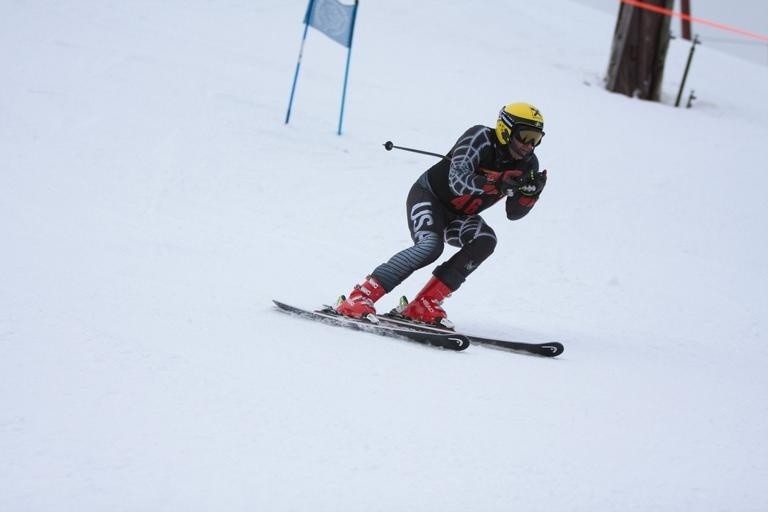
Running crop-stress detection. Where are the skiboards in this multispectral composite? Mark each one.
[272,299,564,357]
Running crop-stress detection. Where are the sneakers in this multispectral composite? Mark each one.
[335,294,379,325]
[401,299,455,330]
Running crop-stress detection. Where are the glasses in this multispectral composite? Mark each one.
[512,123,544,147]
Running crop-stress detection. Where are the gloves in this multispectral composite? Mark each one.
[521,171,548,196]
[494,170,522,198]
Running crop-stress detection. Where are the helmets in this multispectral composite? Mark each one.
[495,102,544,155]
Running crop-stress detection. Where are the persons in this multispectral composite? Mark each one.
[337,101,547,325]
